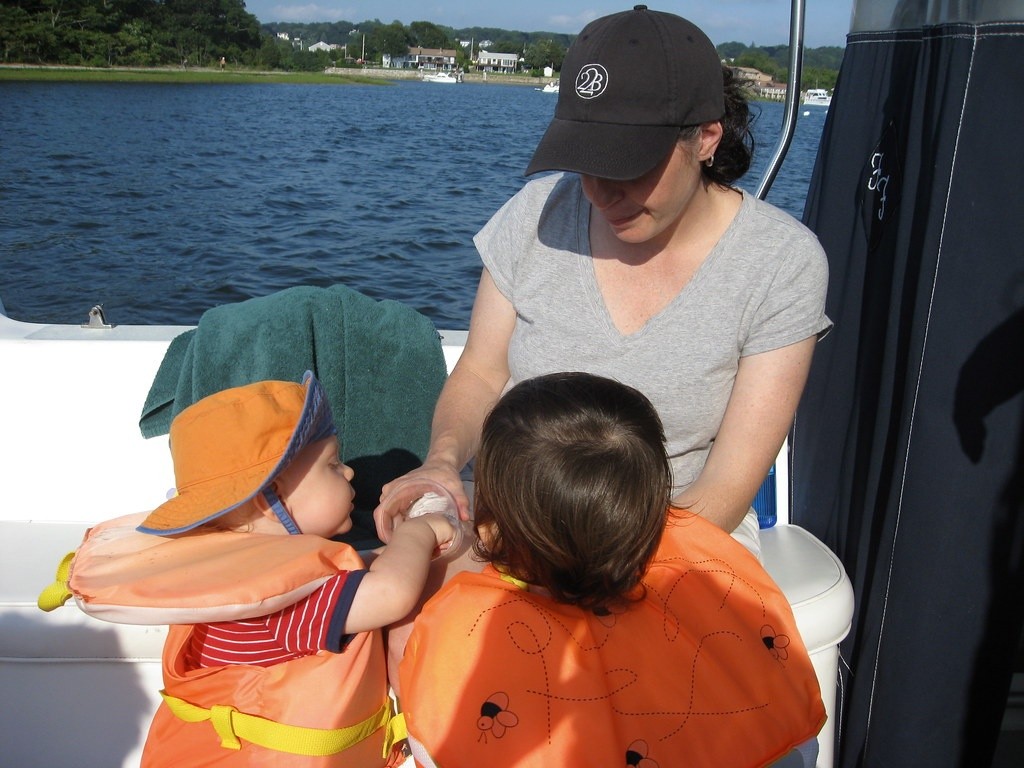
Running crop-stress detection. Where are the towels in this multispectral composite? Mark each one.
[139,285,449,552]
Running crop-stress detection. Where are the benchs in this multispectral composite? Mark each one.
[0,519,855,768]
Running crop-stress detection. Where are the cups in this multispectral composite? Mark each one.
[381,479,463,562]
[750,461,777,529]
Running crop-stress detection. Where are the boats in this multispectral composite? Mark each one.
[804,88,831,106]
[541,81,561,95]
[423,70,458,83]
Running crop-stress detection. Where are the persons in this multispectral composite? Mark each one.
[388,368,828,768]
[36,369,456,768]
[372,4,835,561]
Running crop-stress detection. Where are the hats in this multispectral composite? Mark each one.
[135,370,338,537]
[525,4,726,180]
